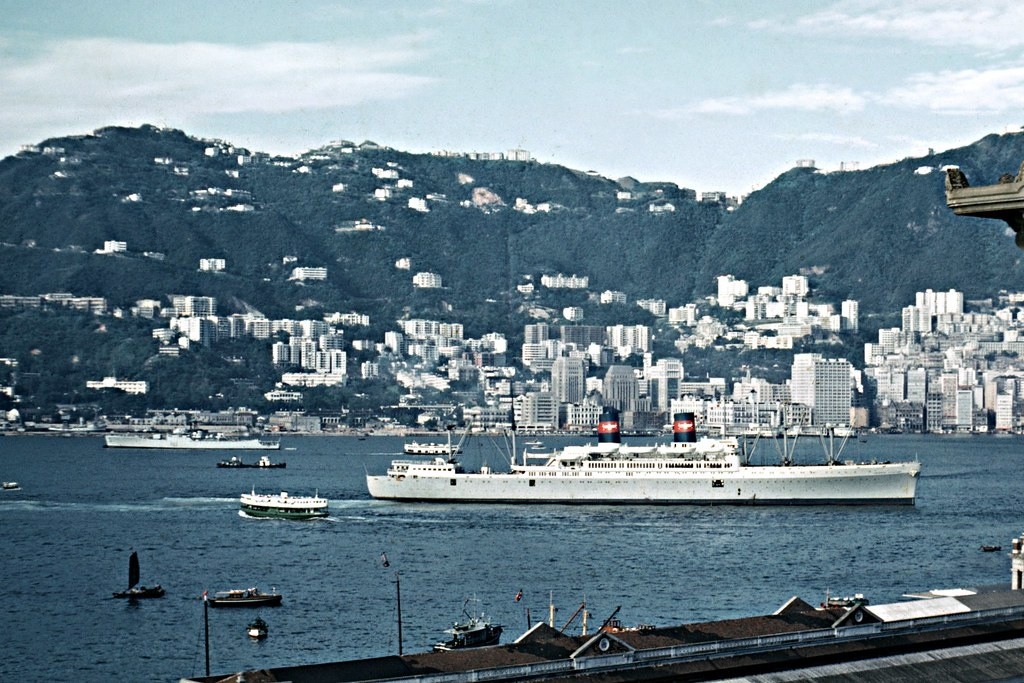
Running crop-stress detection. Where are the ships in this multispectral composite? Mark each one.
[363,406,921,505]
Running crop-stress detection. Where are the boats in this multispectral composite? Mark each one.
[901,588,977,599]
[3,482,19,489]
[696,424,1024,438]
[599,618,655,633]
[404,440,464,455]
[530,445,547,450]
[432,592,506,651]
[240,483,330,520]
[982,544,1003,551]
[247,619,268,637]
[828,593,869,606]
[521,441,544,446]
[208,588,283,606]
[105,418,282,450]
[217,459,286,467]
[589,428,660,437]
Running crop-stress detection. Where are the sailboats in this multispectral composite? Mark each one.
[113,551,165,598]
